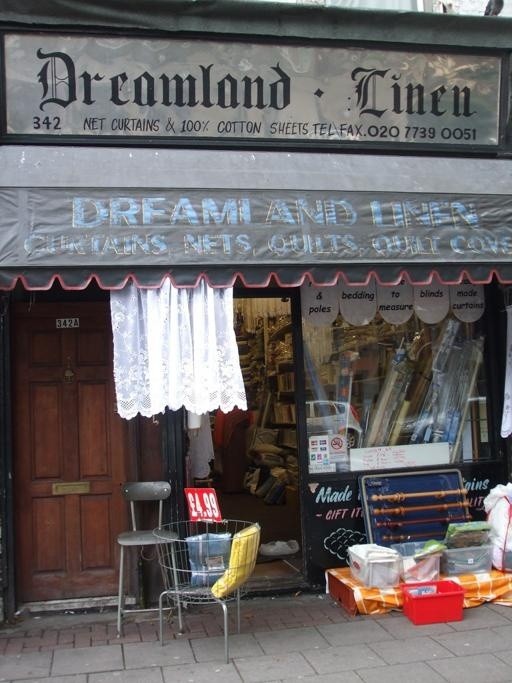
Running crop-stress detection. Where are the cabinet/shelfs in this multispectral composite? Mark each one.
[235,305,494,464]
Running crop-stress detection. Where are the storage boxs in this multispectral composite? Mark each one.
[440,536,493,577]
[347,543,400,589]
[390,541,439,583]
[400,580,466,625]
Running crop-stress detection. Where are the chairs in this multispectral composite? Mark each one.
[116,480,185,637]
